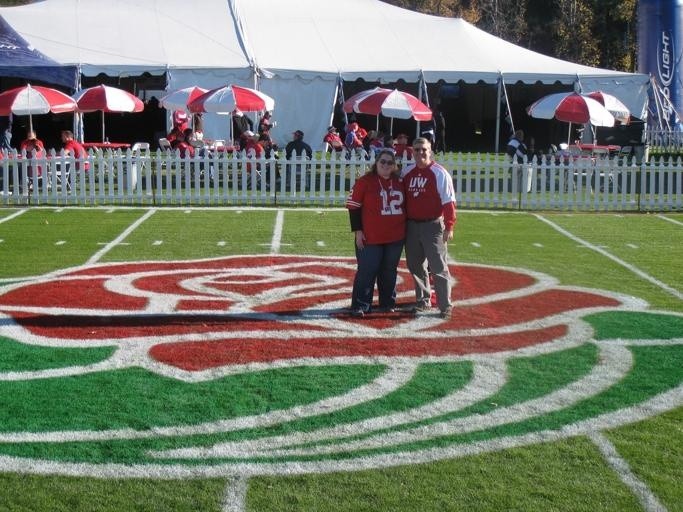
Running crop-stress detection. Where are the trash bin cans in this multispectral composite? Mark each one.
[117,156,142,191]
[510,161,533,193]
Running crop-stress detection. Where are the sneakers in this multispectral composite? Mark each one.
[440,310,451,319]
[382,306,394,313]
[351,307,364,317]
[412,305,431,313]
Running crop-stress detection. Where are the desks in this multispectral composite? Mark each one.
[538,143,620,192]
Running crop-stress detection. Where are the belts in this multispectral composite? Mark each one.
[406,218,436,223]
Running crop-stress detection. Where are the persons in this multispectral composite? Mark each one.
[24,139,42,179]
[402,137,458,320]
[0,120,15,154]
[581,124,597,147]
[230,107,280,189]
[321,121,412,160]
[60,130,90,191]
[20,129,44,150]
[506,129,528,159]
[285,130,313,167]
[347,149,407,320]
[165,111,213,180]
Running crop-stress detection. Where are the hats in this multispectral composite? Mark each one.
[291,130,304,136]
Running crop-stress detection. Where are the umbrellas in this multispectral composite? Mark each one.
[186,83,275,147]
[581,91,631,145]
[524,90,616,150]
[342,86,392,131]
[341,88,433,136]
[157,85,209,135]
[0,84,77,138]
[71,82,145,146]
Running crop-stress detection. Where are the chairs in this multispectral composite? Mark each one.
[613,146,632,166]
[47,137,310,192]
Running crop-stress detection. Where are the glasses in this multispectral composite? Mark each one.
[379,159,393,165]
